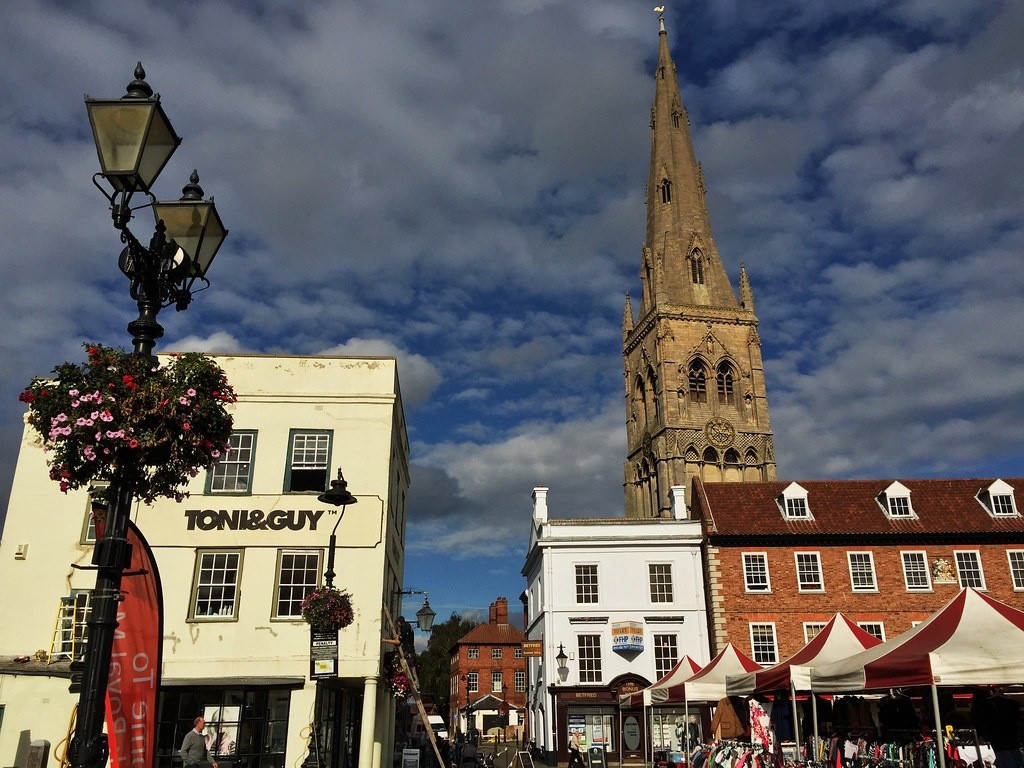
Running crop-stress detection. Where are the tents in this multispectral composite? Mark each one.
[619,586,1024,768]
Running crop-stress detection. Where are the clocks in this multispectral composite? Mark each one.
[704,416,736,449]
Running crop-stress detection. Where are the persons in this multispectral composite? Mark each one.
[180,717,219,768]
[675,714,699,753]
[398,616,414,657]
[745,398,752,424]
[568,731,587,768]
[707,338,714,355]
[411,726,480,768]
[678,393,685,419]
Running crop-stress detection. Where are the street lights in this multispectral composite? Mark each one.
[460,673,470,742]
[302,468,358,768]
[502,683,509,743]
[58,56,232,768]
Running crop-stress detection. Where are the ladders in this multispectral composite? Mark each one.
[47,597,77,665]
[384,603,446,768]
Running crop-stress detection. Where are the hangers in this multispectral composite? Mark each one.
[717,738,773,758]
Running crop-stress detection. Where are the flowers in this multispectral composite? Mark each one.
[377,672,412,702]
[16,339,238,505]
[299,585,356,633]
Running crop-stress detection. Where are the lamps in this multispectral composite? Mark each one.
[556,640,569,668]
[393,585,437,632]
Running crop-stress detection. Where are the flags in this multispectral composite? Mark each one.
[92,502,164,768]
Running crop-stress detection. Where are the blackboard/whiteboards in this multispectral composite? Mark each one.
[515,751,534,768]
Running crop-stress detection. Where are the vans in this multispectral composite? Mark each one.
[411,714,449,742]
